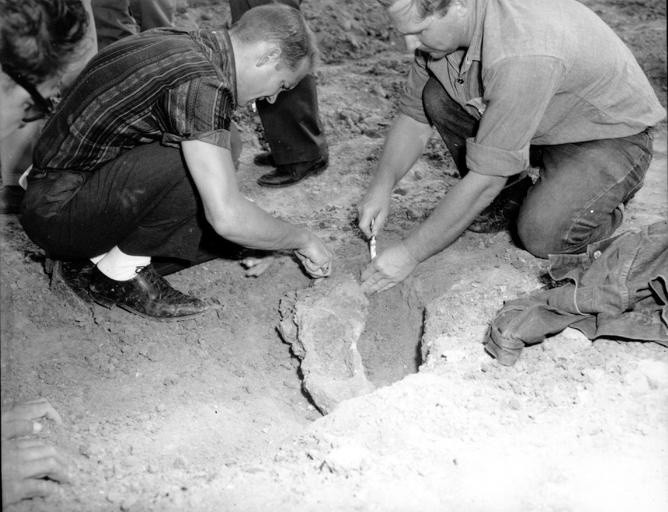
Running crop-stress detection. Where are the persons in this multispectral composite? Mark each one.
[230,0,331,188]
[0,0,177,212]
[353,0,668,299]
[16,2,337,321]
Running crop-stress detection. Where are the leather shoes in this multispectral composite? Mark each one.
[254,149,329,188]
[86,262,210,322]
